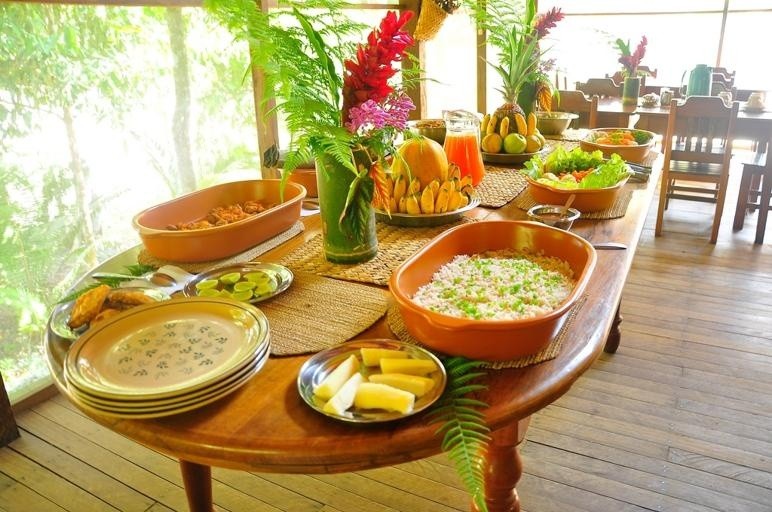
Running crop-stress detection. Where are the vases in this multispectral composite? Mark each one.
[516,82,538,124]
[313,146,379,266]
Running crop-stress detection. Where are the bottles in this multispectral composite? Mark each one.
[660,90,671,106]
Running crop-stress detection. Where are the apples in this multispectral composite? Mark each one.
[482,129,546,154]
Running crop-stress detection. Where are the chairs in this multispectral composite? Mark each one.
[550,66,772,245]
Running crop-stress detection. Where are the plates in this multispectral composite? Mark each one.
[64,300,271,422]
[49,287,175,343]
[367,189,483,226]
[480,143,549,165]
[294,337,452,424]
[180,263,302,303]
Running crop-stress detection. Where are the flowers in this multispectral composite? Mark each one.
[612,34,657,80]
[461,0,566,118]
[201,0,451,250]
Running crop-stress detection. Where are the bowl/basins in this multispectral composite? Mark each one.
[534,110,580,136]
[407,119,456,143]
[526,203,582,233]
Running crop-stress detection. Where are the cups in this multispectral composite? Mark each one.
[716,90,767,110]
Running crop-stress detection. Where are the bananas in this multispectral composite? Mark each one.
[377,162,474,215]
[481,113,537,138]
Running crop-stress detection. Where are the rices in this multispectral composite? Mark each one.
[407,247,577,320]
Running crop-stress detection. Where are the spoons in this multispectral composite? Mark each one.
[90,270,178,286]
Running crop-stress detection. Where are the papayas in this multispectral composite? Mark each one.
[391,136,449,192]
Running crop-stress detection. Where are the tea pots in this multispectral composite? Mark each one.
[680,64,713,99]
[441,109,486,188]
